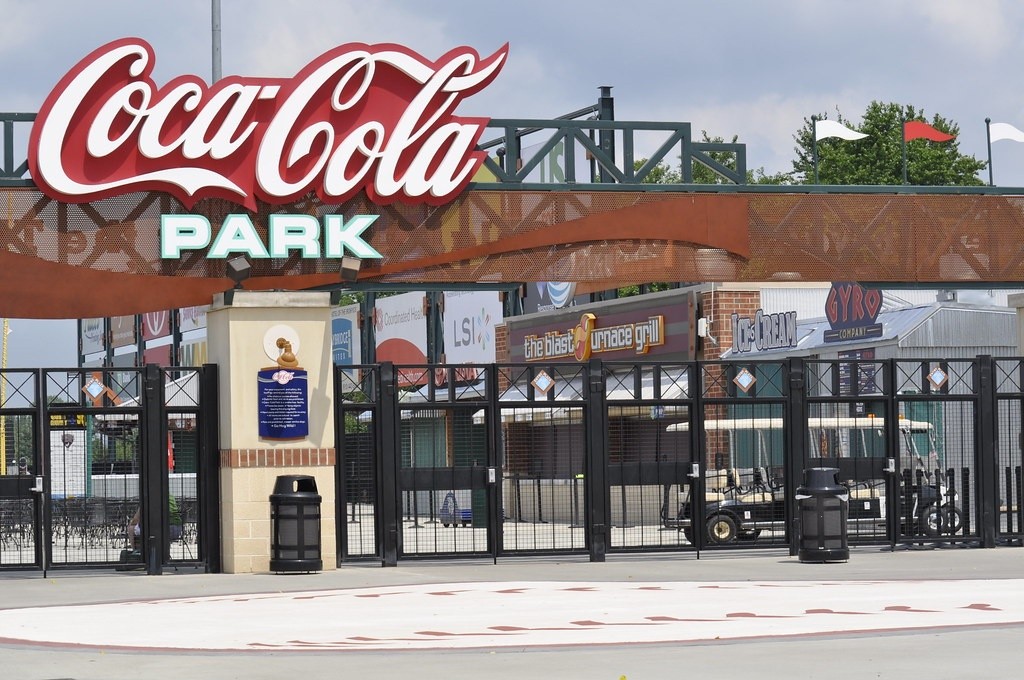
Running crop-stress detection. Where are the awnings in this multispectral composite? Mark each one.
[355,381,485,422]
[472,365,705,425]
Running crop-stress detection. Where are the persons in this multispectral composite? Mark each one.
[127,496,182,551]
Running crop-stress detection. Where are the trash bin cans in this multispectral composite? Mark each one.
[795,467,850,563]
[269,474,323,575]
